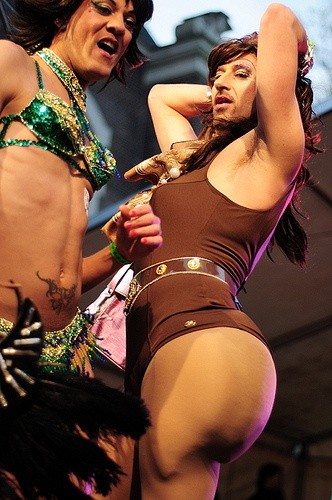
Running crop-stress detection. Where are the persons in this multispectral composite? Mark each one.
[0,0,163,500]
[123,3,314,500]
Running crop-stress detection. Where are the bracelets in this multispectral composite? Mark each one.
[301,40,315,63]
[109,241,134,266]
